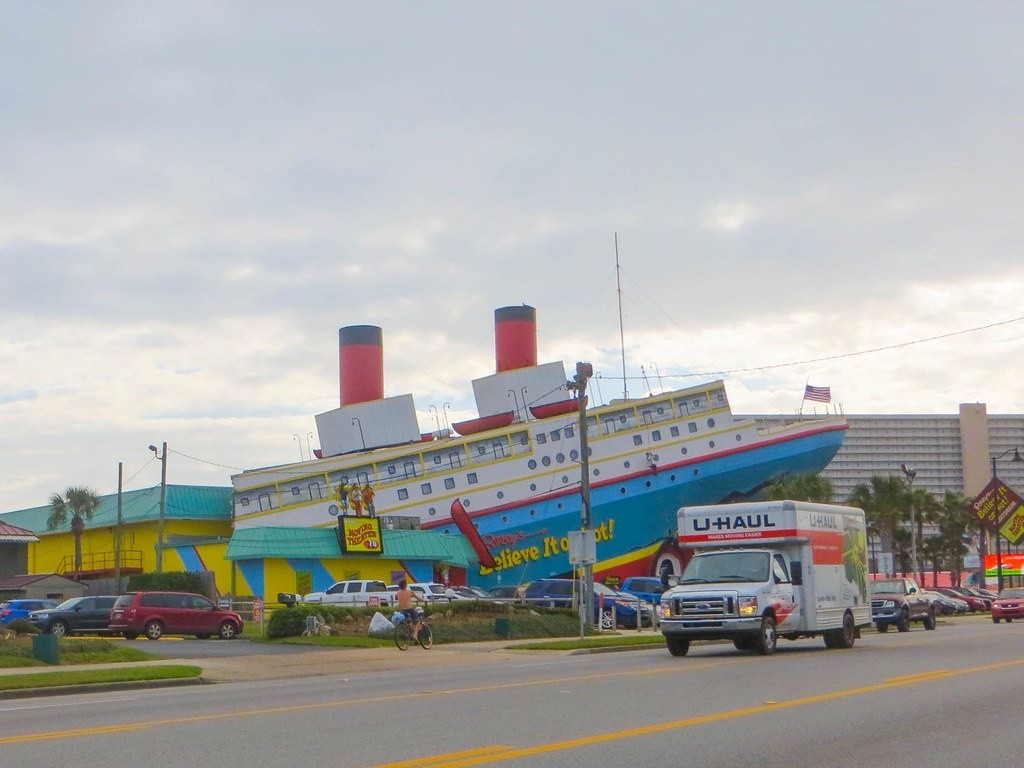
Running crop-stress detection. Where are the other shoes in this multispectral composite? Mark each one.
[411,634,417,640]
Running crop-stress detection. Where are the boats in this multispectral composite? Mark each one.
[226,304,853,606]
[420,432,435,442]
[450,409,517,436]
[527,394,590,421]
[312,449,322,459]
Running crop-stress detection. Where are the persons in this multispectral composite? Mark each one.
[395,580,426,642]
[363,484,376,518]
[352,485,363,517]
[340,483,348,516]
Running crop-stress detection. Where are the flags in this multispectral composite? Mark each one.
[804,385,830,403]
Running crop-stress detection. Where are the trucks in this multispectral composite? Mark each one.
[659,500,873,657]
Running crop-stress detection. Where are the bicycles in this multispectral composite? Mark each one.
[392,600,434,651]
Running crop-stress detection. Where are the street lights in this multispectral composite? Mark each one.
[351,416,367,453]
[594,371,605,407]
[506,386,531,423]
[293,431,315,461]
[147,441,168,571]
[990,445,1024,593]
[901,462,917,582]
[639,361,663,396]
[428,402,452,440]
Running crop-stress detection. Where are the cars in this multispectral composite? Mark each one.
[525,578,651,632]
[449,585,503,605]
[106,590,244,639]
[866,577,942,633]
[991,587,1024,624]
[0,599,58,630]
[27,595,122,637]
[921,586,998,616]
[623,577,667,607]
[488,585,522,600]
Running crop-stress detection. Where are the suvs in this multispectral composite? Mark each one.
[387,582,451,605]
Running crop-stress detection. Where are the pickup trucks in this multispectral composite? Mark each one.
[304,578,400,607]
